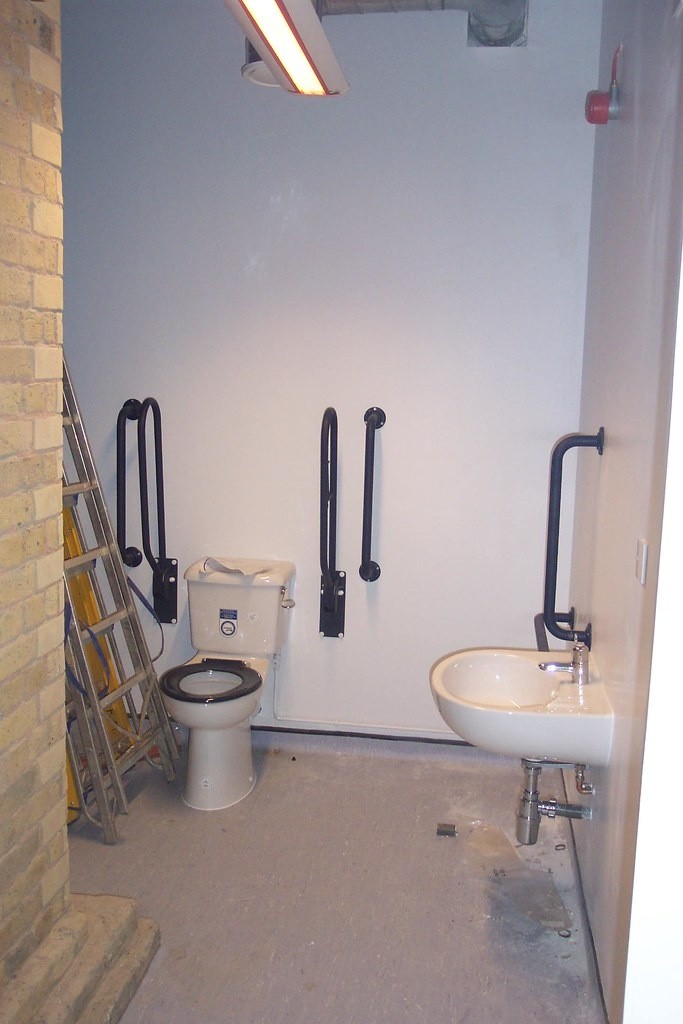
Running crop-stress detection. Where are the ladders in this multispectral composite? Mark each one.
[62,349,186,842]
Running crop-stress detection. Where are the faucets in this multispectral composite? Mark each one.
[538,644,590,685]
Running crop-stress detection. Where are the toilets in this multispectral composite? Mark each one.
[158,555,297,812]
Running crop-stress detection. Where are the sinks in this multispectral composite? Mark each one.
[429,646,617,768]
